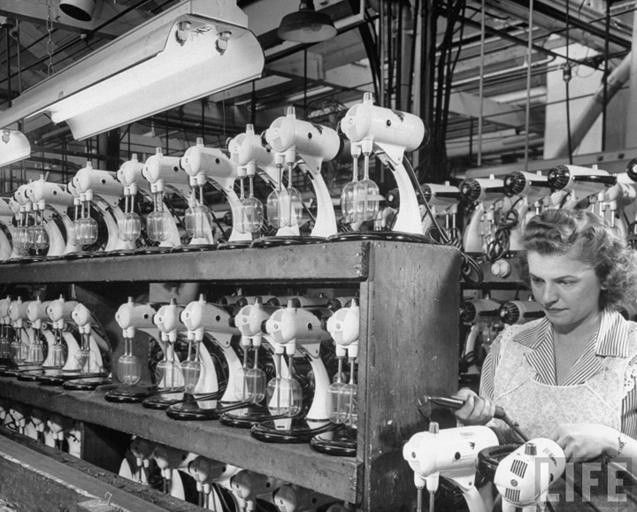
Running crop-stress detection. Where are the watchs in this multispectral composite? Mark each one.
[610,432,625,459]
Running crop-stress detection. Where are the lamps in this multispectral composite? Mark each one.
[0,0,267,144]
[0,101,31,168]
[277,0,337,44]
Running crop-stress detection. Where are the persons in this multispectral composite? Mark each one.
[450,209,637,478]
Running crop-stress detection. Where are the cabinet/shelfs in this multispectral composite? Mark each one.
[0,242,463,512]
